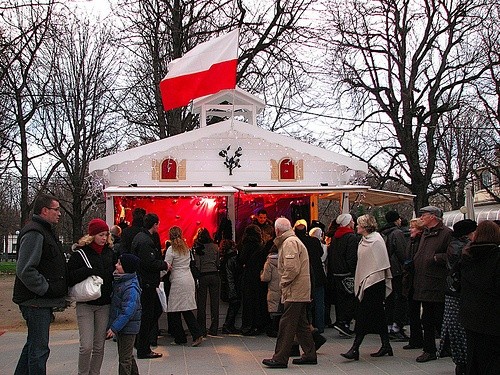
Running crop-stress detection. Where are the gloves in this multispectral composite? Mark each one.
[84,268,99,278]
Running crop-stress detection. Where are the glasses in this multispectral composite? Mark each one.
[41,205,59,211]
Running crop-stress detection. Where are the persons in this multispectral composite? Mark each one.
[340,214,393,360]
[236,210,425,349]
[106,255,142,375]
[193,226,220,337]
[12,193,69,375]
[130,213,172,359]
[220,238,241,334]
[436,219,500,375]
[110,208,205,347]
[412,206,453,362]
[262,217,326,368]
[66,219,116,375]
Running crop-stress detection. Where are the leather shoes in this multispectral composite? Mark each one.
[292,358,317,364]
[137,351,162,359]
[262,357,286,368]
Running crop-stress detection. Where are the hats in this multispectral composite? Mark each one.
[419,206,441,218]
[386,210,400,222]
[89,219,110,236]
[337,213,352,227]
[119,254,141,274]
[453,219,478,237]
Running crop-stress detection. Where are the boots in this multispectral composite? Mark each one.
[313,328,327,352]
[222,315,239,334]
[370,331,394,357]
[289,343,300,357]
[340,333,364,361]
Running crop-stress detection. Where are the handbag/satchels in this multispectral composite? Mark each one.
[190,260,199,281]
[65,275,104,302]
[444,266,463,295]
[328,265,356,306]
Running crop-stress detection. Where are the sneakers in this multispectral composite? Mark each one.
[332,321,355,337]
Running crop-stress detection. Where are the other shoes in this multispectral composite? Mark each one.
[192,335,208,346]
[207,329,217,336]
[389,330,409,341]
[416,351,437,363]
[403,343,423,349]
[171,342,184,346]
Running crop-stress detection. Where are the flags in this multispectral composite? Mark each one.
[159,29,239,111]
